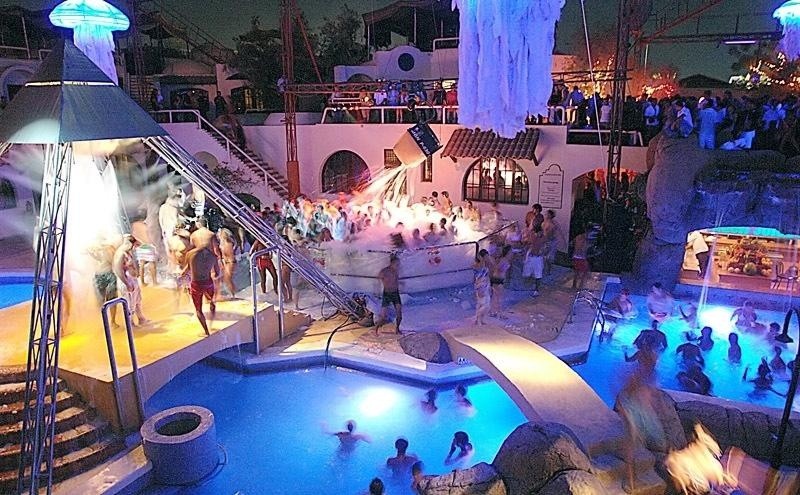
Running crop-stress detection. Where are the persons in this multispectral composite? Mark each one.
[599,282,800,401]
[149,87,228,132]
[326,78,459,126]
[548,80,799,154]
[44,250,84,331]
[329,383,473,495]
[94,195,592,336]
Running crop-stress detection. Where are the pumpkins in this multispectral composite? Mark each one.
[743,263,757,275]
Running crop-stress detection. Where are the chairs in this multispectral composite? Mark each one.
[772,261,798,290]
